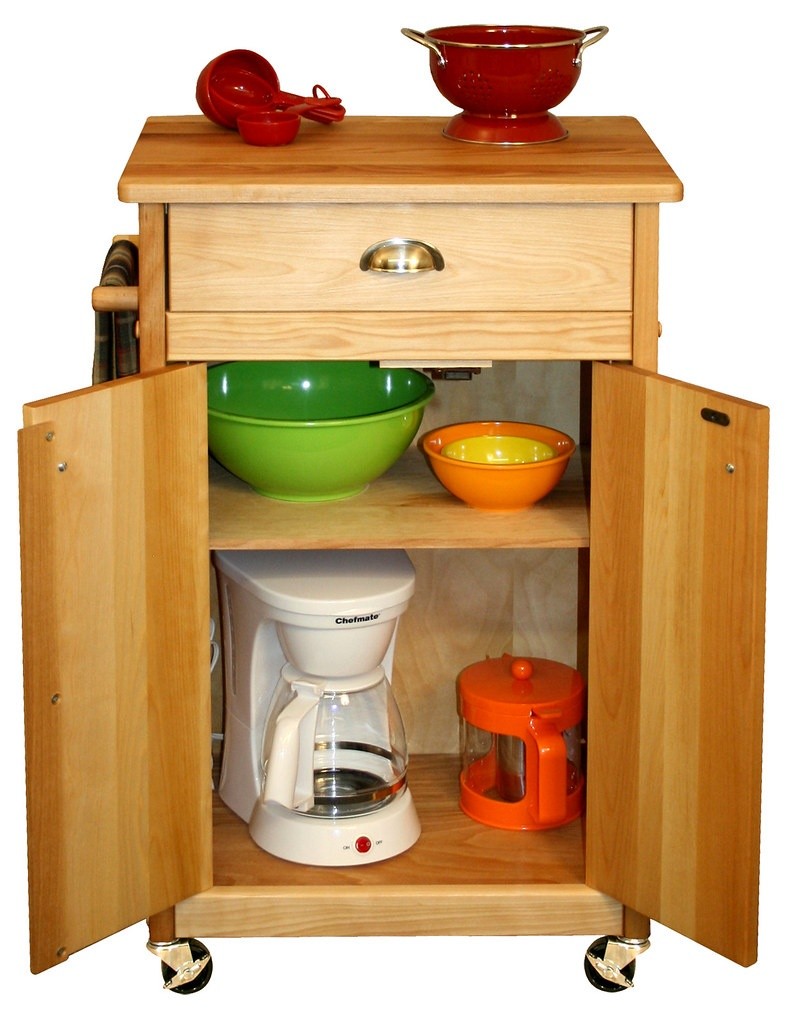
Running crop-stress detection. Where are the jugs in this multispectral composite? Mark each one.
[456,655,587,830]
[257,662,409,815]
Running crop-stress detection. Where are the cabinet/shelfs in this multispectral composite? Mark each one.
[16,116,769,991]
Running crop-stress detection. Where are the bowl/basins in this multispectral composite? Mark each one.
[238,110,301,146]
[418,421,574,511]
[196,49,285,128]
[207,361,435,503]
[400,25,607,145]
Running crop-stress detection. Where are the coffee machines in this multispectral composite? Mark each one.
[209,546,418,869]
